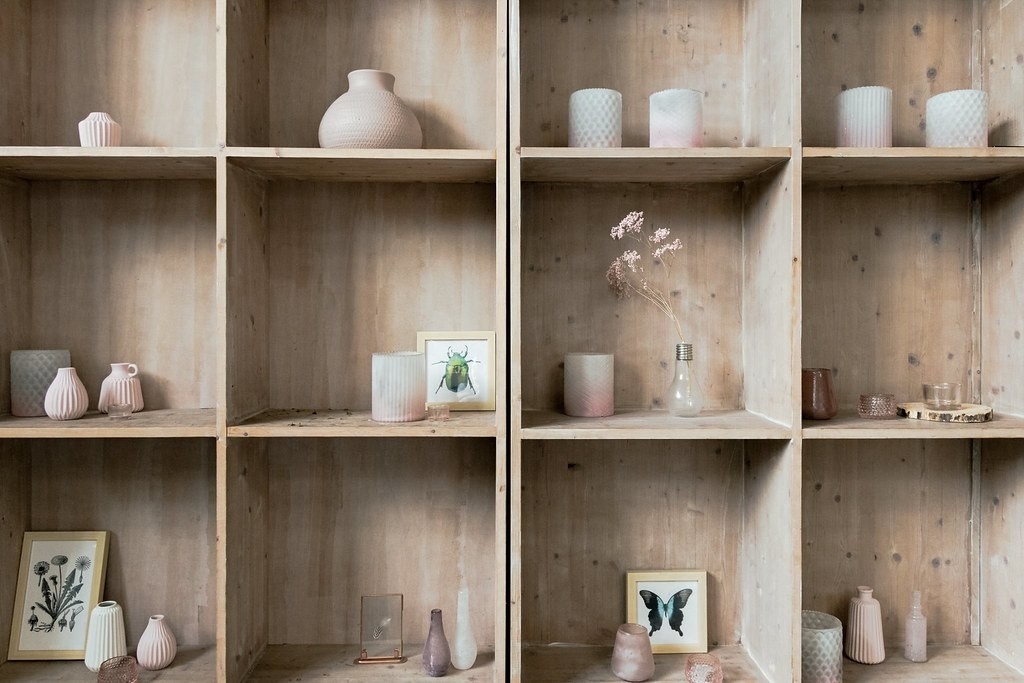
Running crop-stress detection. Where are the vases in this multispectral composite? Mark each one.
[318,70,423,149]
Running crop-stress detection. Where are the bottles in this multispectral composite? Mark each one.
[903,591,928,662]
[450,590,478,672]
[421,607,452,677]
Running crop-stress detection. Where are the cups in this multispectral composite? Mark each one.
[684,653,724,683]
[922,382,962,410]
[107,403,133,420]
[925,90,991,148]
[562,352,615,417]
[371,351,427,422]
[835,85,893,147]
[649,88,704,148]
[97,656,139,683]
[568,87,623,148]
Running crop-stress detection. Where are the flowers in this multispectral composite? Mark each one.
[606,212,684,343]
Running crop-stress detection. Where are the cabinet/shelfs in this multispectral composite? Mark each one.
[0,0,1024,683]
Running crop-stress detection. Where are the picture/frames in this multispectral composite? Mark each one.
[625,569,707,654]
[416,331,496,411]
[7,531,111,660]
